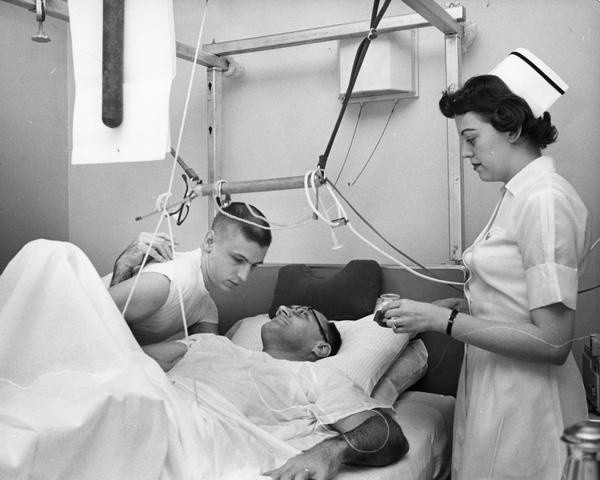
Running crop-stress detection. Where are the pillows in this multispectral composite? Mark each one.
[372,339,430,404]
[229,312,410,396]
[269,259,385,321]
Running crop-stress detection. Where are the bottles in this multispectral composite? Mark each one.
[558,420,599,480]
[374,292,401,328]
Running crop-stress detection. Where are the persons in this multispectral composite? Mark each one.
[108,232,410,480]
[380,45,589,480]
[101,201,271,347]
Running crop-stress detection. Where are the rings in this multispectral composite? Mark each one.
[304,466,311,476]
[392,320,400,330]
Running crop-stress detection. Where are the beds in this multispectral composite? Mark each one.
[198,256,469,480]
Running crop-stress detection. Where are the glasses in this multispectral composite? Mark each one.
[271,305,328,341]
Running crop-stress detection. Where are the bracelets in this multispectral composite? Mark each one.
[446,310,458,336]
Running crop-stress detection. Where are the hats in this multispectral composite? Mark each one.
[487,47,569,120]
